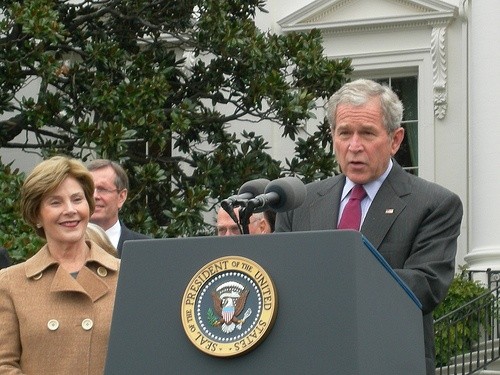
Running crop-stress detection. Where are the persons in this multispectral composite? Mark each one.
[84,222,119,259]
[215,203,276,236]
[0,156,121,375]
[271,78,465,375]
[85,159,150,257]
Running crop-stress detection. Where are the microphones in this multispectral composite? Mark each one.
[241,176,307,223]
[221,178,271,211]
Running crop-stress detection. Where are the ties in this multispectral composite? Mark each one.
[338,185,368,231]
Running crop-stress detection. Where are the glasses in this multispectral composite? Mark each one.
[217,221,253,236]
[95,186,118,193]
